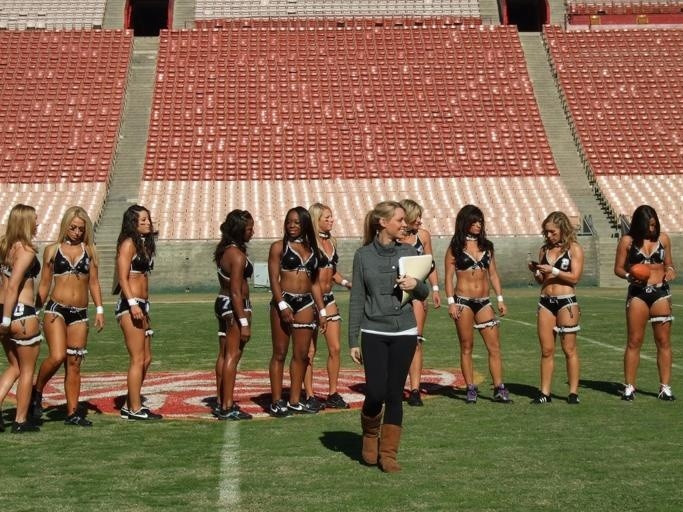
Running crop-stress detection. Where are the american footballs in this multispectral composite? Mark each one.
[629,264,651,281]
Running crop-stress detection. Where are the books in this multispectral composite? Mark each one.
[395,248,433,308]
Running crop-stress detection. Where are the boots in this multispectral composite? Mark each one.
[361,410,400,472]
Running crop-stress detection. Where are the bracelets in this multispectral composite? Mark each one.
[550,266,560,278]
[33,306,41,316]
[340,279,348,287]
[319,307,327,317]
[277,300,289,312]
[94,304,105,315]
[431,284,439,293]
[239,315,249,328]
[446,295,455,305]
[1,315,13,327]
[495,293,503,304]
[533,268,540,278]
[624,272,630,281]
[667,265,674,269]
[127,297,137,306]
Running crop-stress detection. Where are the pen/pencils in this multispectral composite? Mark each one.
[393,273,407,289]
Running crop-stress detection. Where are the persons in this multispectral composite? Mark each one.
[111,203,165,420]
[526,211,584,406]
[612,204,678,400]
[400,198,441,407]
[1,205,45,435]
[210,209,255,420]
[348,201,433,473]
[31,206,105,426]
[444,204,516,404]
[304,202,352,411]
[267,206,329,418]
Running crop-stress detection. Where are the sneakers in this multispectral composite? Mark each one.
[659,386,677,402]
[270,393,350,416]
[121,406,162,421]
[494,385,514,403]
[11,421,39,435]
[213,403,252,420]
[466,384,478,403]
[408,389,423,407]
[530,391,551,405]
[65,413,93,426]
[622,383,636,402]
[568,394,580,405]
[29,387,43,418]
